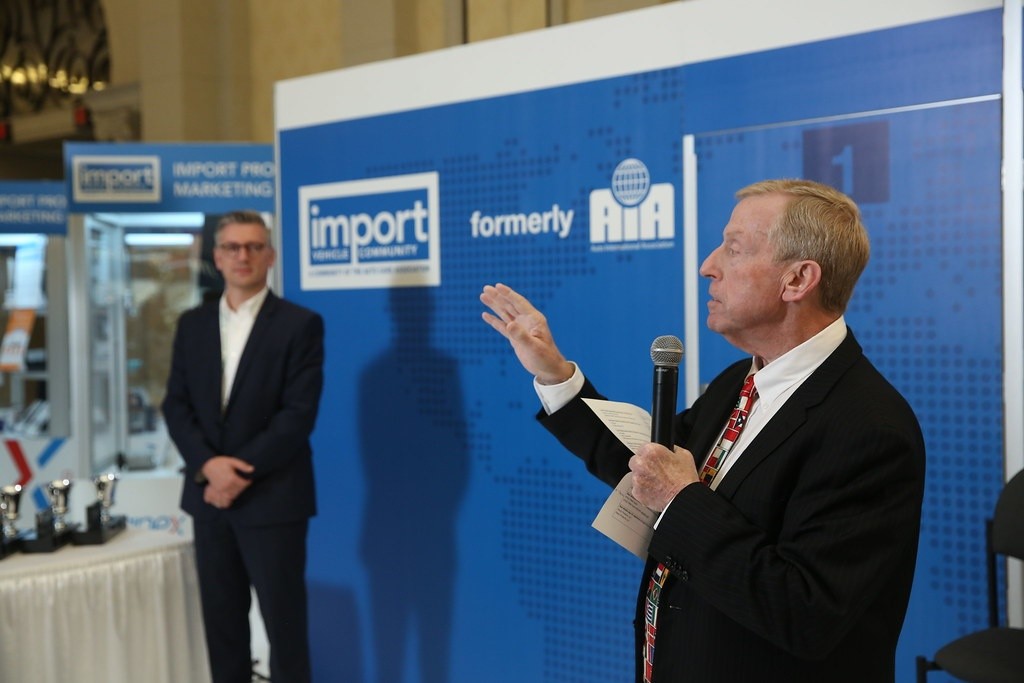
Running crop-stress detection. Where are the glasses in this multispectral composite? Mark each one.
[215,240,270,260]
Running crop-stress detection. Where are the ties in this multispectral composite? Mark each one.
[643,373,760,683]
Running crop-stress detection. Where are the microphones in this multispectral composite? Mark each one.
[650,334,685,453]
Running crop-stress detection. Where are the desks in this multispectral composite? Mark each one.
[0,475,212,683]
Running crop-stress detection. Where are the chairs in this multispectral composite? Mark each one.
[915,468,1024,683]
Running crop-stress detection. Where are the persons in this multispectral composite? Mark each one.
[478,179,928,683]
[160,212,326,683]
